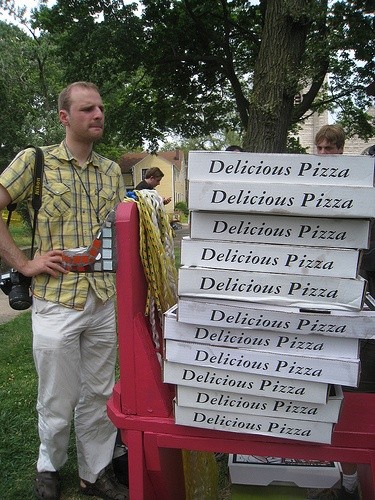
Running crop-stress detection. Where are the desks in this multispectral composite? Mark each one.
[119,429,375,500]
[104,193,375,449]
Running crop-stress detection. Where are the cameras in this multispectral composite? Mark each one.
[0,267,33,310]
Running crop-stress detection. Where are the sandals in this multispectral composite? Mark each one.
[78,467,129,500]
[34,470,62,500]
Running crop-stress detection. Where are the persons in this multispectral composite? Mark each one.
[315,124,345,154]
[135,166,172,206]
[0,81,127,500]
[315,462,360,500]
[225,145,243,153]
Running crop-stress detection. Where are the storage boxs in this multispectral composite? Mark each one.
[158,152,375,490]
